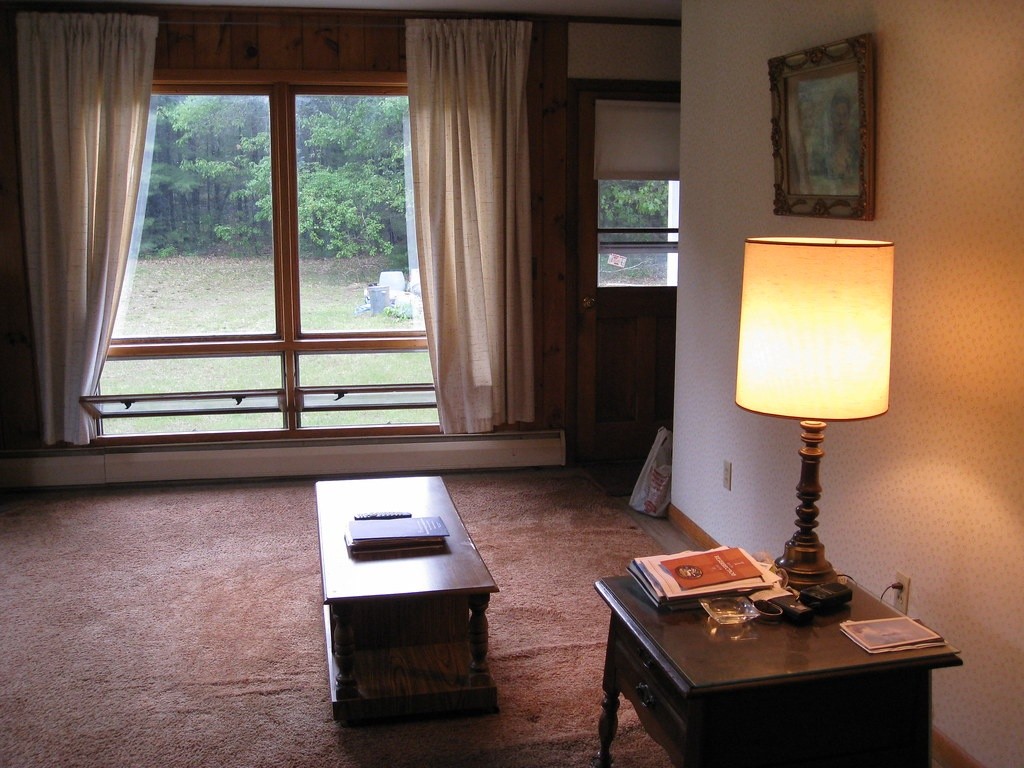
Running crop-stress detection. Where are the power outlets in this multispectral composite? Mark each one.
[893,571,910,616]
[723,459,732,491]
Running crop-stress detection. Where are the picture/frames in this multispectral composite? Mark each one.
[768,33,875,222]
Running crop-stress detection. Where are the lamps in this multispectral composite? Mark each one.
[736,236,894,592]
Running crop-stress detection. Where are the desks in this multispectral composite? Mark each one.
[594,565,963,768]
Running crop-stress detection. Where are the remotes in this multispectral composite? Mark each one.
[354,513,412,521]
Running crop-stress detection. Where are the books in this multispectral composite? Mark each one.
[840,617,949,654]
[625,545,782,611]
[344,516,450,555]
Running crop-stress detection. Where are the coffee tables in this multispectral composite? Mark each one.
[315,475,502,724]
